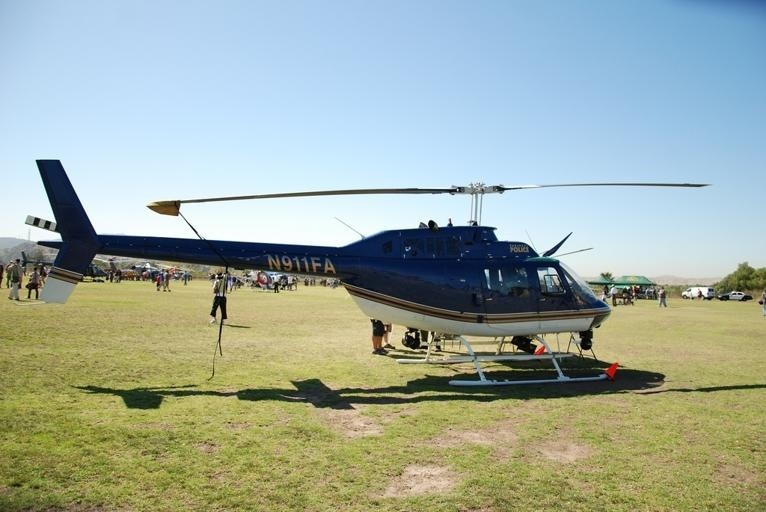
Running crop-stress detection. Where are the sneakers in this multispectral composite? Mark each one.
[372,347,389,355]
[209,319,217,324]
[419,345,428,349]
[14,298,19,301]
[7,297,13,300]
[434,348,443,352]
[221,320,224,325]
[383,343,396,349]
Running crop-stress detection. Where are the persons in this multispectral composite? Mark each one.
[37,262,48,288]
[92,265,345,294]
[208,267,228,325]
[420,328,442,353]
[658,286,668,307]
[369,317,390,356]
[762,289,766,316]
[27,267,39,300]
[0,258,24,301]
[602,284,657,308]
[698,289,702,298]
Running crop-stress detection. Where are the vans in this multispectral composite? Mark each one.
[682,287,715,299]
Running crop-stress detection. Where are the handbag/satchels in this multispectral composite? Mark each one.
[214,286,220,293]
[11,275,20,283]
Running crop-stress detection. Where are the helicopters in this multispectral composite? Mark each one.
[20,251,107,283]
[154,263,196,281]
[24,157,711,386]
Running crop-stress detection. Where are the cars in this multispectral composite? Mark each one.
[717,291,754,302]
